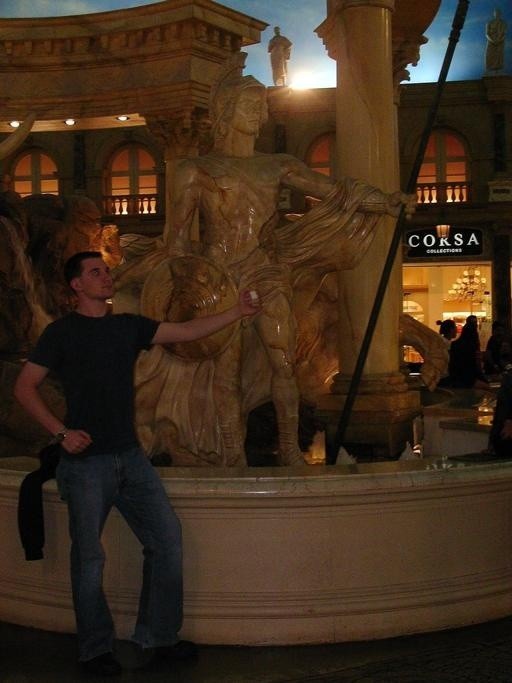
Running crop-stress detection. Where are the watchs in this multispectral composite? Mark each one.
[55,429,70,441]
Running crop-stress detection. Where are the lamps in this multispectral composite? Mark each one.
[432,216,454,238]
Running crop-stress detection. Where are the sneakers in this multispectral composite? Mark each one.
[138,641,196,659]
[81,653,127,682]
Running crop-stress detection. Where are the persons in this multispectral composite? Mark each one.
[437,319,458,341]
[13,252,263,642]
[483,321,512,376]
[165,76,418,469]
[267,26,294,88]
[486,7,507,77]
[457,315,481,356]
